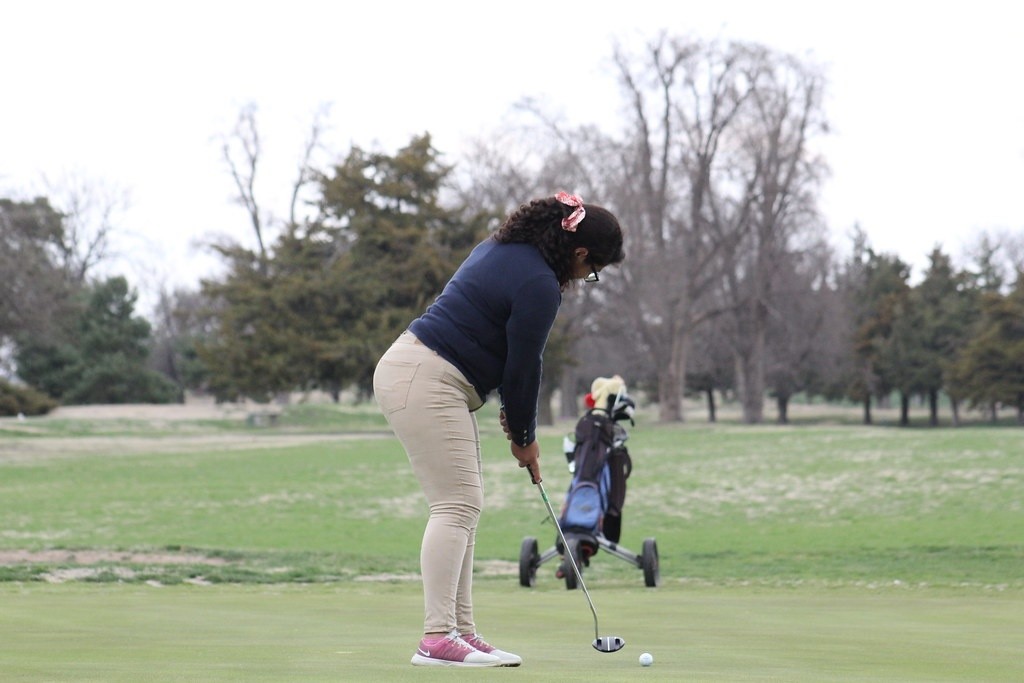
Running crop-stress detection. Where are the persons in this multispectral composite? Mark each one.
[373,190,628,670]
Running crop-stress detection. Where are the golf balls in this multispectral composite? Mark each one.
[639,653,653,666]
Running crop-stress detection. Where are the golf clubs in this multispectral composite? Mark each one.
[499,404,625,653]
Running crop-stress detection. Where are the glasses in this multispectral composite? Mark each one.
[584,262,599,283]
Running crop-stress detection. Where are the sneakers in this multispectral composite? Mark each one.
[409,633,522,668]
